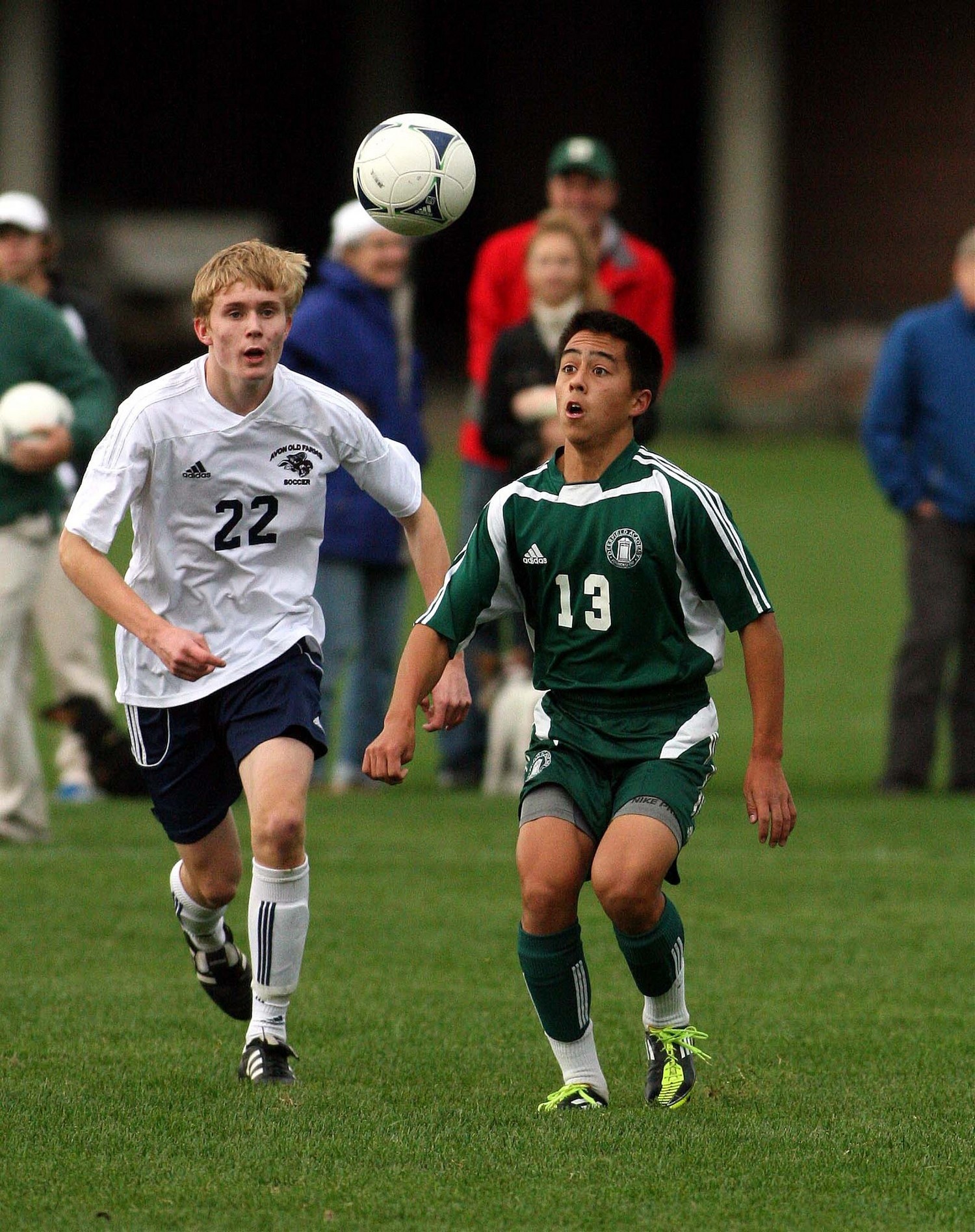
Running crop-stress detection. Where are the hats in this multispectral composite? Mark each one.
[1,191,50,237]
[548,138,612,183]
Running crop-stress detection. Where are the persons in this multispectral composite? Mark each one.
[862,225,975,795]
[60,236,474,1087]
[480,221,657,800]
[432,136,672,792]
[0,187,113,844]
[277,197,430,795]
[362,311,797,1110]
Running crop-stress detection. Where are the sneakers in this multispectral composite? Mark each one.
[537,1083,608,1111]
[642,1023,713,1110]
[237,1036,300,1087]
[183,922,253,1022]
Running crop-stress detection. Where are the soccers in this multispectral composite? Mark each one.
[351,107,476,242]
[1,383,74,463]
